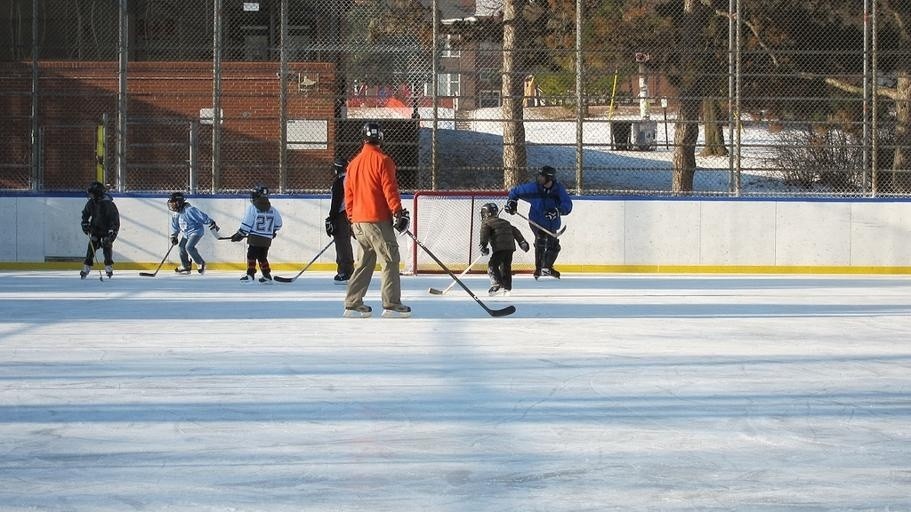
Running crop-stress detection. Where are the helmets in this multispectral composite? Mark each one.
[536,165,555,185]
[86,183,107,199]
[481,203,498,217]
[361,123,384,144]
[167,193,186,211]
[333,159,346,172]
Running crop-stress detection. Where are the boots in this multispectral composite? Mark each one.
[81,265,91,275]
[106,265,113,273]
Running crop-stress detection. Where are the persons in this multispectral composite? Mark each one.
[166,192,222,273]
[231,183,283,281]
[507,164,574,279]
[480,202,532,293]
[327,157,356,282]
[340,123,417,315]
[81,181,120,276]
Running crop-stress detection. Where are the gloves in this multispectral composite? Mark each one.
[520,242,529,252]
[326,219,334,236]
[393,208,411,233]
[108,231,116,242]
[210,222,219,231]
[232,233,243,242]
[170,237,179,244]
[479,244,489,255]
[544,207,560,221]
[81,221,91,235]
[506,198,517,215]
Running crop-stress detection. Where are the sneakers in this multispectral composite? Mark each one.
[386,304,411,312]
[335,273,350,280]
[347,305,371,313]
[174,265,191,273]
[260,275,272,281]
[197,262,205,272]
[240,275,253,280]
[541,267,559,277]
